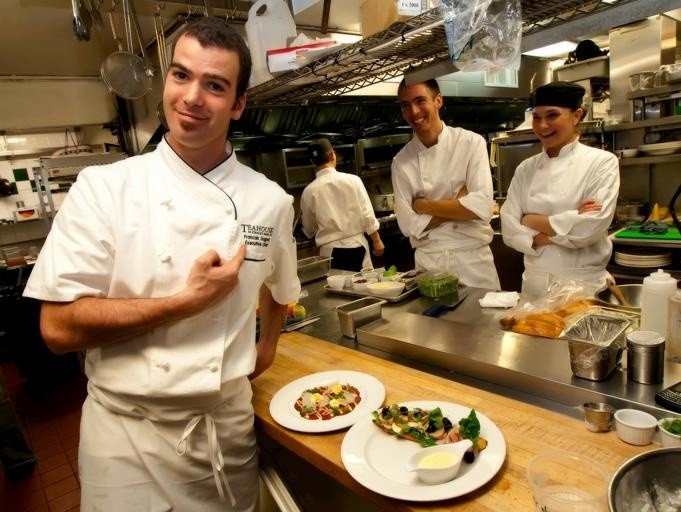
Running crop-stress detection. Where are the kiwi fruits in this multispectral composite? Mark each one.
[293,305,305,320]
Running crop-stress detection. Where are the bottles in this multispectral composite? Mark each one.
[624,384,661,402]
[626,331,665,384]
[640,266,681,378]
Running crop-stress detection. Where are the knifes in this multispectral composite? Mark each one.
[423,285,473,316]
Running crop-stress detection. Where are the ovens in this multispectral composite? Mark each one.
[355,134,418,177]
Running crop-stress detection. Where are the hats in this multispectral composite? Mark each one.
[308,138,332,153]
[530,81,586,108]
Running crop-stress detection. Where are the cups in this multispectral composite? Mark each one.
[526,451,610,512]
[583,402,614,432]
[660,418,680,446]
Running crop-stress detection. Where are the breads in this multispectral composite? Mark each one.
[511,311,567,339]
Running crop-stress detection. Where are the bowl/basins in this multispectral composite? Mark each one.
[596,283,642,310]
[615,407,659,447]
[607,447,679,512]
[628,71,656,90]
[615,201,651,225]
[326,265,421,299]
[615,147,638,158]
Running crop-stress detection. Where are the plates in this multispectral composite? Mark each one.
[266,367,388,436]
[638,141,681,155]
[613,248,673,268]
[340,401,506,502]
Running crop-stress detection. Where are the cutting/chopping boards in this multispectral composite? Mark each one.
[616,223,681,239]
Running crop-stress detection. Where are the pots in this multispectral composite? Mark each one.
[633,102,665,121]
[370,192,395,212]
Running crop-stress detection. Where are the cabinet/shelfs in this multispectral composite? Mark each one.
[262,143,359,190]
[601,83,680,166]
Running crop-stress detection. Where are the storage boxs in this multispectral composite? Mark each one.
[357,0,444,38]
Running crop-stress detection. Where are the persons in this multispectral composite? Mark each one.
[22,14,303,512]
[499,80,620,301]
[300,138,385,271]
[390,68,501,291]
